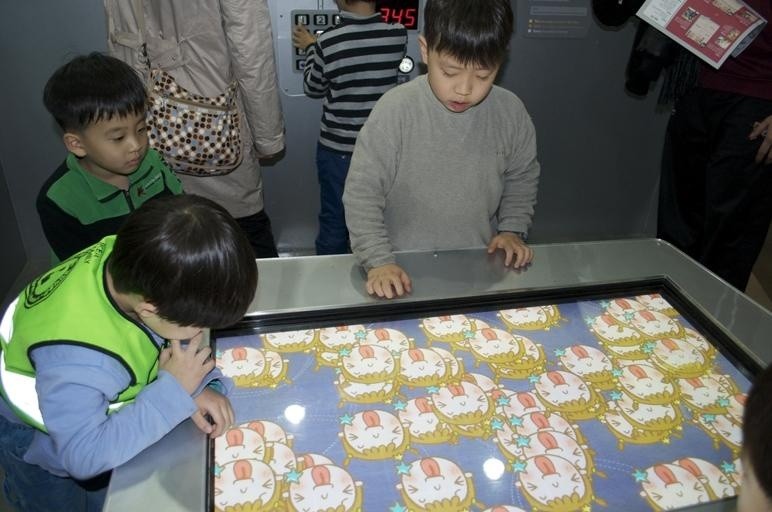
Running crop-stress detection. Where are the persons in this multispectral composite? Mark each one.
[292,0,407,256]
[734,361,771,512]
[0,193,258,511]
[341,0,542,300]
[105,0,289,258]
[656,1,772,293]
[35,49,185,262]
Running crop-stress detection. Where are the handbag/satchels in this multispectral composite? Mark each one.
[144,67,244,177]
